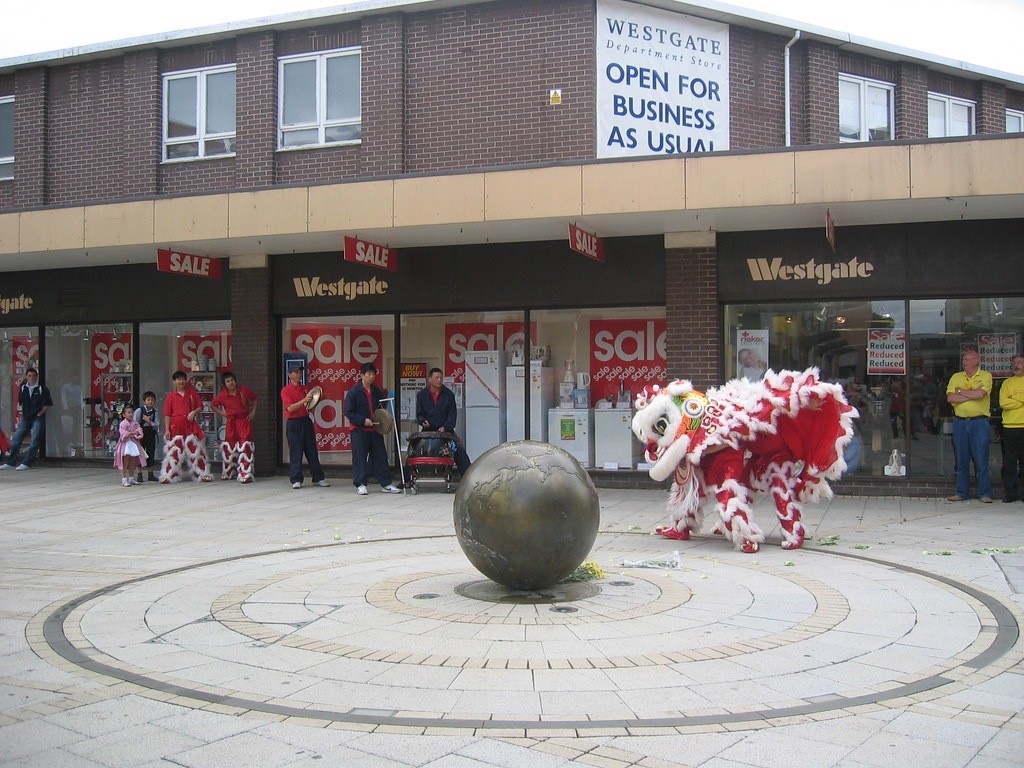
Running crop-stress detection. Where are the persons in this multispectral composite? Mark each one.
[999,355,1024,503]
[738,349,765,382]
[211,372,258,484]
[281,366,329,487]
[396,368,471,489]
[133,391,160,482]
[885,376,952,440]
[0,368,53,470]
[946,350,993,503]
[159,371,213,483]
[344,363,402,495]
[114,407,147,486]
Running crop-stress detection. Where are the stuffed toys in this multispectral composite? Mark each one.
[632,367,859,552]
[828,378,872,422]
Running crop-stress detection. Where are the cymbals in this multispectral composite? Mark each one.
[305,385,323,409]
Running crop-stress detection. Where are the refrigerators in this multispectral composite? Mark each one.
[506,366,553,444]
[595,408,644,469]
[464,350,507,464]
[548,408,595,468]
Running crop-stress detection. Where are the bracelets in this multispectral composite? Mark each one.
[957,389,960,394]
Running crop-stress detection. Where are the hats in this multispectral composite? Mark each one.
[288,365,305,373]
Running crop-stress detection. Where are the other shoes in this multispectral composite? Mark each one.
[396,482,403,489]
[1002,496,1017,502]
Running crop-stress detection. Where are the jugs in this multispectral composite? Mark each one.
[576,372,590,390]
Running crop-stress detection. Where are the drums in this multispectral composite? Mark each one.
[405,427,458,478]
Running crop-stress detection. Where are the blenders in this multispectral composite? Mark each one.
[561,358,574,383]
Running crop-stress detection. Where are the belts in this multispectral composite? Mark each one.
[957,416,988,420]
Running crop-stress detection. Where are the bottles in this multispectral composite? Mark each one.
[209,359,216,372]
[198,355,208,372]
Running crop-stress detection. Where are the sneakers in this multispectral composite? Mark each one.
[948,495,968,500]
[137,477,143,481]
[313,479,330,486]
[15,464,30,470]
[292,481,303,488]
[148,476,159,481]
[981,497,992,502]
[0,464,16,470]
[129,479,140,484]
[356,484,368,494]
[381,484,401,493]
[121,480,130,486]
